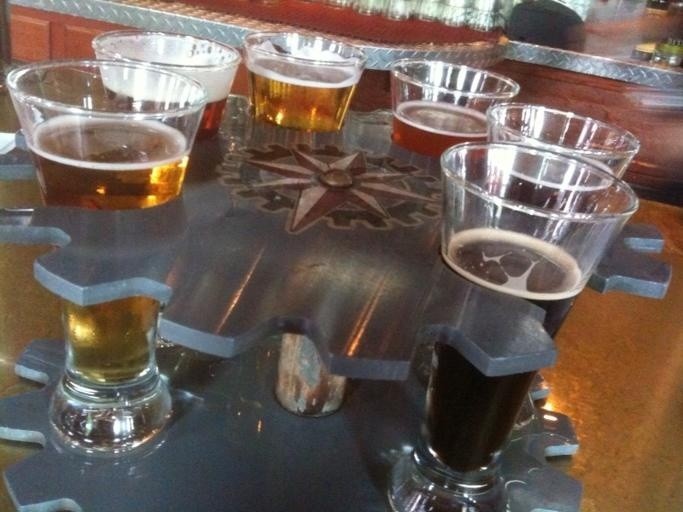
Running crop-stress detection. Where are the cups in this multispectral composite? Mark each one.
[487,101,641,253]
[243,31,369,135]
[390,59,521,157]
[649,0,682,66]
[93,30,242,135]
[382,141,640,512]
[6,62,209,457]
